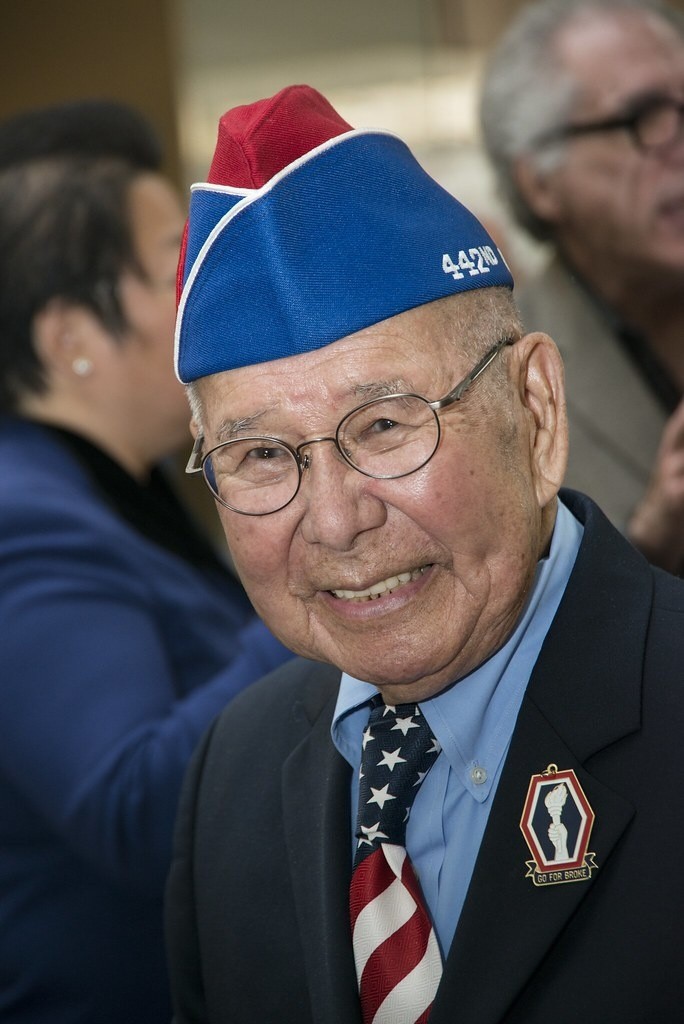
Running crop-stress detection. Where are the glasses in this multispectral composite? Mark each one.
[530,90,684,165]
[184,337,517,517]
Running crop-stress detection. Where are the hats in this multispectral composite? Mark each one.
[173,84,514,382]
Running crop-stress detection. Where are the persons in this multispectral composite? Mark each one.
[164,84,684,1024]
[0,99,307,1024]
[477,0,684,577]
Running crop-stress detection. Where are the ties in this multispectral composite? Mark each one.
[347,697,446,1024]
